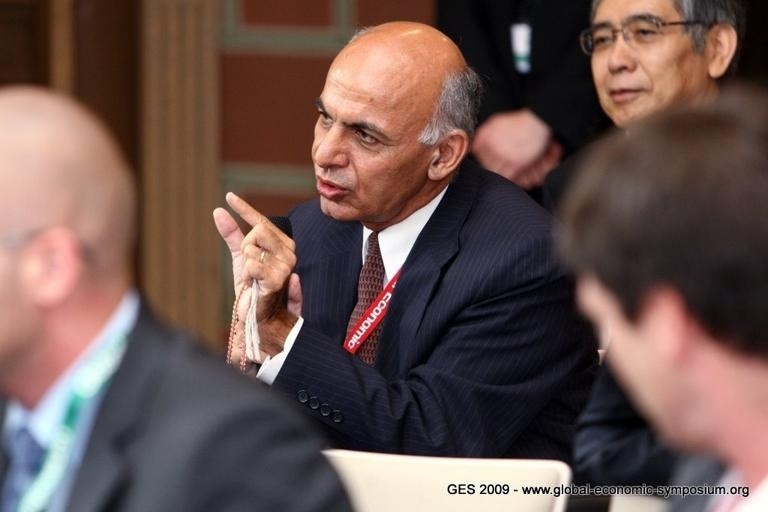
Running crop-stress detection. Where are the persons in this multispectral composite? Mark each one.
[432,1,617,217]
[567,1,768,512]
[211,19,602,461]
[0,82,353,512]
[550,87,768,512]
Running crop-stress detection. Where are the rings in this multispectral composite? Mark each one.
[259,249,266,262]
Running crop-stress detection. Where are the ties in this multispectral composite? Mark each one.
[342,231,387,366]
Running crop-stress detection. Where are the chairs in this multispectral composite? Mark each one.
[320,448,572,511]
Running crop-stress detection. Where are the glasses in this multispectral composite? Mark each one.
[580,15,702,55]
